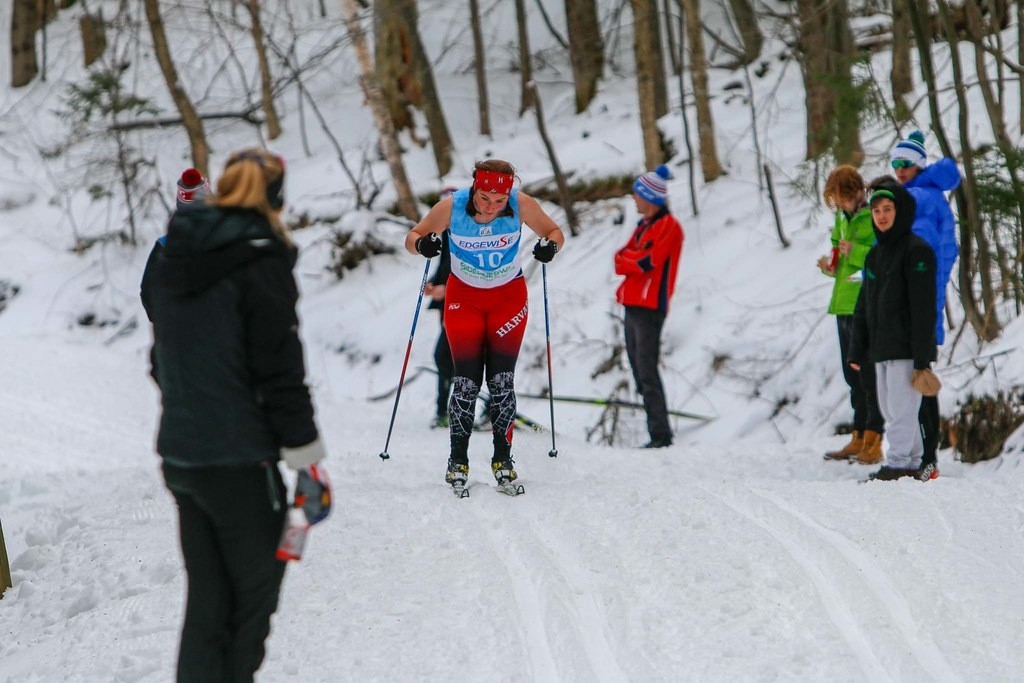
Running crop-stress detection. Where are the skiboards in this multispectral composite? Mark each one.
[451,478,526,499]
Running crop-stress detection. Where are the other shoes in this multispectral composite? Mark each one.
[913,462,939,481]
[864,462,918,481]
[430,418,450,429]
[647,438,671,448]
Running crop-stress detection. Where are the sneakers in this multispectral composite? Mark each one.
[491,461,517,485]
[444,453,469,483]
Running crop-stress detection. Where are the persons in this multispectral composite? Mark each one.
[816,164,878,435]
[613,164,684,448]
[405,159,565,487]
[865,174,897,197]
[141,168,214,387]
[141,145,326,683]
[846,182,939,482]
[422,188,493,431]
[893,129,961,479]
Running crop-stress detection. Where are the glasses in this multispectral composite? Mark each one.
[892,159,915,171]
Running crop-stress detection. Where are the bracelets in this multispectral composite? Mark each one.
[549,241,558,253]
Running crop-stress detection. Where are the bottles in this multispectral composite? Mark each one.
[275,495,310,563]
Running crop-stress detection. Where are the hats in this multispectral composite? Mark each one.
[176,168,210,209]
[892,130,928,171]
[632,165,669,207]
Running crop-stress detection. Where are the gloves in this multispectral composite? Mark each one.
[277,459,313,561]
[532,239,556,263]
[414,233,441,258]
[296,461,331,525]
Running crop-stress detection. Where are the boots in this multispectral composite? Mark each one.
[849,429,885,465]
[823,429,864,461]
